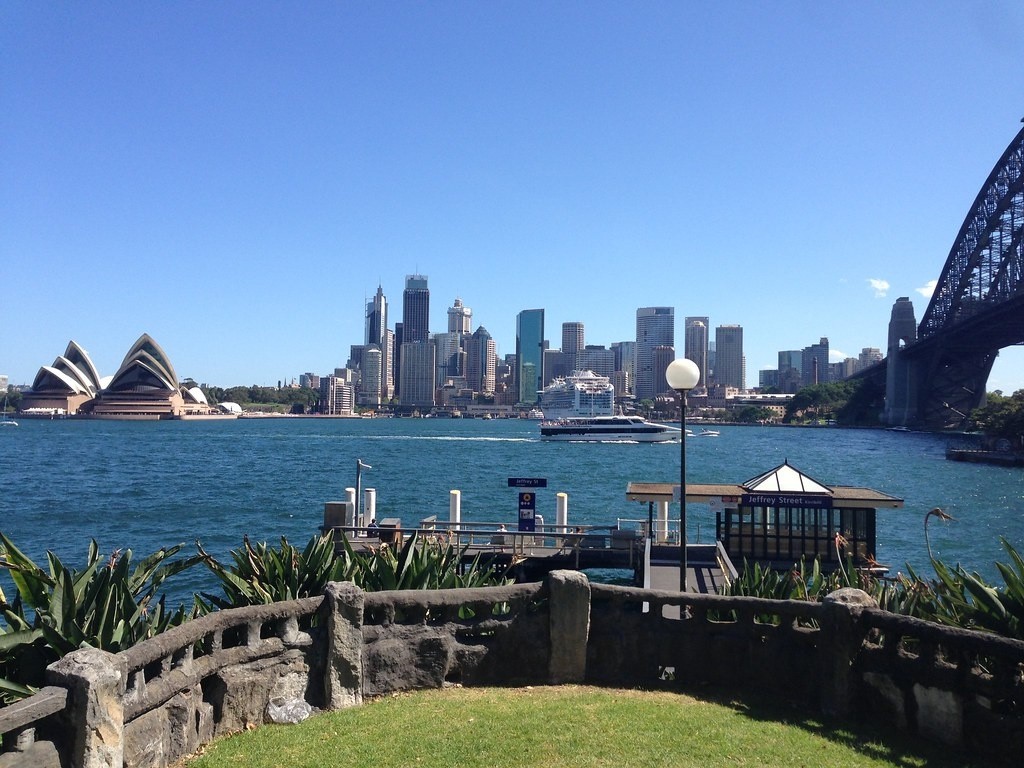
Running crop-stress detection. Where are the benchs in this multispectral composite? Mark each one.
[564,538,606,554]
[491,535,535,555]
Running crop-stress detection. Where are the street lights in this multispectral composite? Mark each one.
[665,357,700,620]
[354,461,371,536]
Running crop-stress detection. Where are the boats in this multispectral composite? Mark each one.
[528,409,544,420]
[886,426,911,433]
[482,413,492,420]
[538,404,693,442]
[450,408,460,418]
[538,369,614,420]
[699,428,720,436]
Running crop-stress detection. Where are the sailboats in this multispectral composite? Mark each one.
[0,395,18,426]
